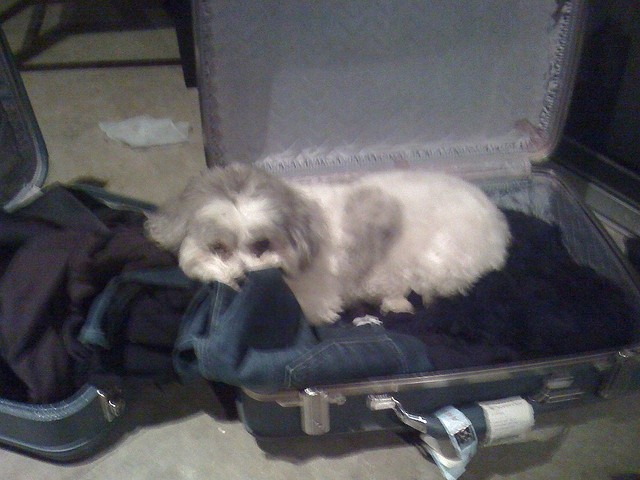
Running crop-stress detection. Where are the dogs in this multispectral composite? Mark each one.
[142,159,513,327]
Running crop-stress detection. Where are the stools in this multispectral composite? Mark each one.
[0,1,196,88]
